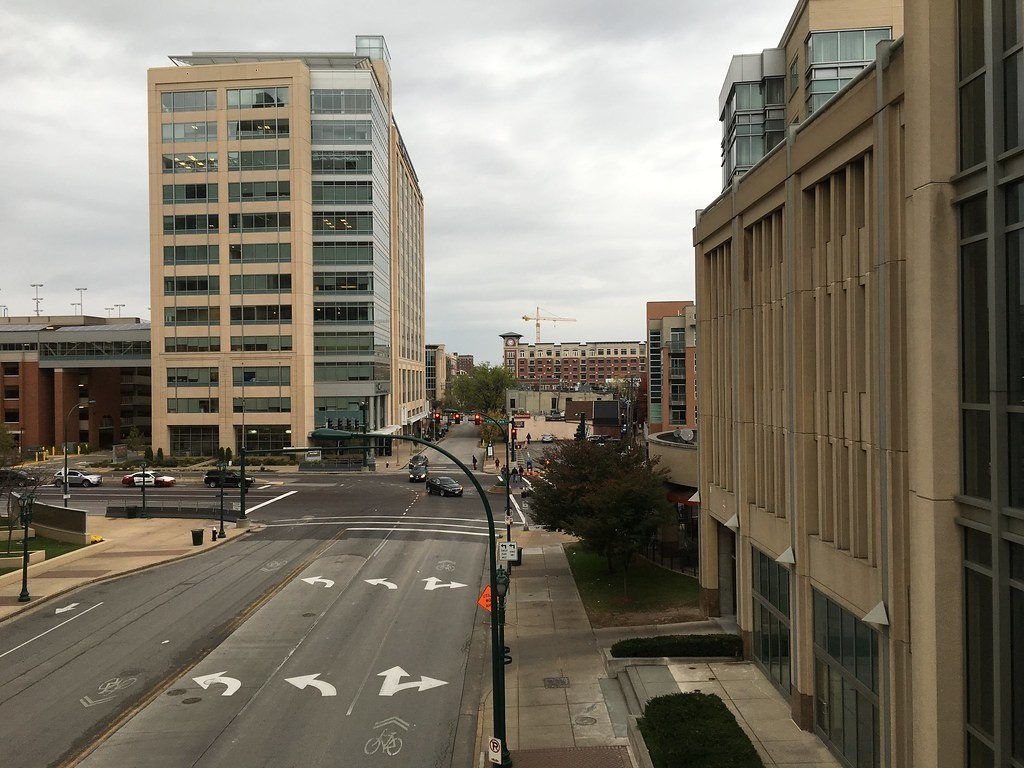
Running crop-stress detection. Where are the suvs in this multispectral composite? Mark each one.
[203,470,254,488]
[52,469,103,488]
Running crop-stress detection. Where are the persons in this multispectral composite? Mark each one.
[501,465,524,480]
[526,433,531,444]
[471,455,478,471]
[494,457,501,470]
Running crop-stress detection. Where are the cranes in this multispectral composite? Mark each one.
[522,306,578,344]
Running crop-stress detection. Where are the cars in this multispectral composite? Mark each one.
[438,428,445,438]
[541,433,554,443]
[409,456,428,469]
[426,476,464,497]
[468,416,475,421]
[121,471,176,487]
[443,425,448,431]
[408,467,429,482]
[586,435,621,447]
[0,469,39,487]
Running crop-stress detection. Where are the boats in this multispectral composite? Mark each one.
[516,445,521,449]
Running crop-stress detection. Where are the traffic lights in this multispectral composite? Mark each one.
[435,412,441,423]
[475,414,480,425]
[455,414,460,424]
[512,429,516,440]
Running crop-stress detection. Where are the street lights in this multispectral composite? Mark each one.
[140,462,148,517]
[64,401,96,507]
[0,283,125,320]
[17,493,37,602]
[495,566,511,666]
[307,427,514,768]
[216,459,227,537]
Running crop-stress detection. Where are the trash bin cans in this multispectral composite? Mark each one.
[511,547,523,566]
[190,528,204,546]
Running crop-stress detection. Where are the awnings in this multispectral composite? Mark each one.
[405,411,432,424]
[369,425,402,435]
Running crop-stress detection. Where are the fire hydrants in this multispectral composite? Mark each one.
[385,462,389,468]
[212,527,217,541]
